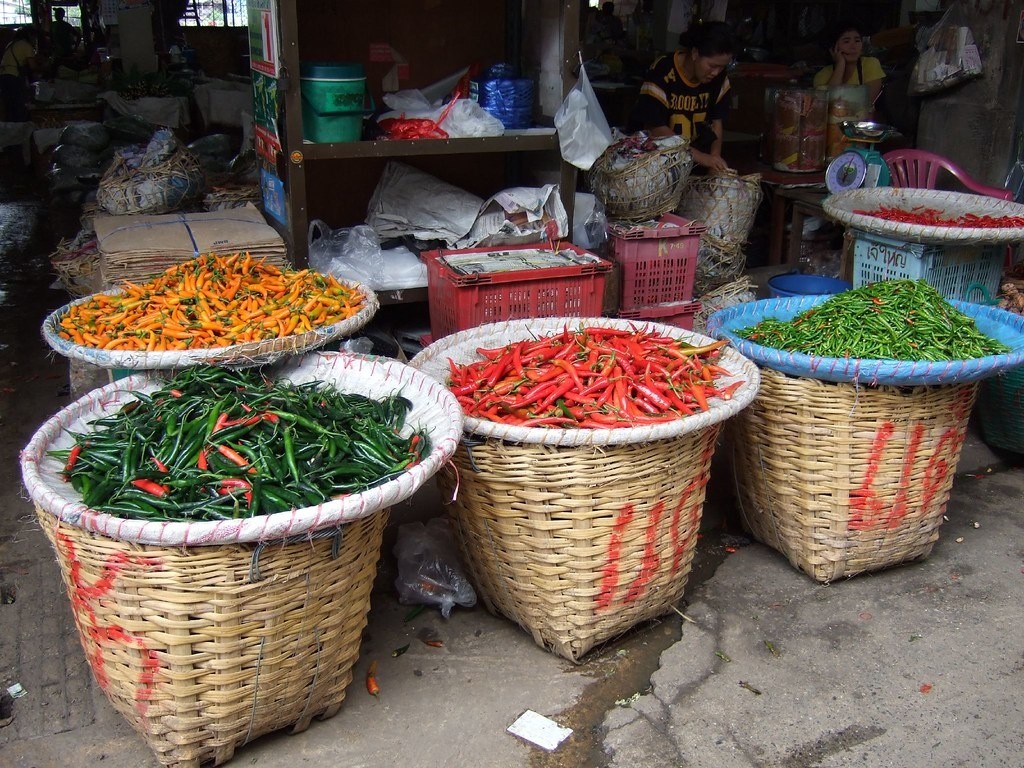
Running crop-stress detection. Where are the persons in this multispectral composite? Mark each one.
[51,8,79,56]
[631,21,738,176]
[814,27,886,103]
[602,2,623,47]
[0,27,46,122]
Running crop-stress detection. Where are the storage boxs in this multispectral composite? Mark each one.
[208,81,248,124]
[419,215,708,350]
[850,229,1008,305]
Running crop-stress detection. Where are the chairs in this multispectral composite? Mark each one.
[883,148,1015,265]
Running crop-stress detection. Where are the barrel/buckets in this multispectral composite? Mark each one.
[756,268,852,301]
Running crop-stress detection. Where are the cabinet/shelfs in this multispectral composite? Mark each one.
[249,0,580,307]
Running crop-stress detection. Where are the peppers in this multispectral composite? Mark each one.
[444,322,746,429]
[45,365,434,520]
[731,277,1014,361]
[852,207,1024,228]
[54,253,366,351]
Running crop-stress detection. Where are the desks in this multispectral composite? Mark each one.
[734,160,824,266]
[787,195,854,281]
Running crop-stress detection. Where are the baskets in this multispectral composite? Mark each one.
[982,363,1024,452]
[434,420,728,665]
[33,503,388,768]
[723,363,981,585]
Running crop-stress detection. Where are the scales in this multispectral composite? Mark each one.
[825,119,895,210]
